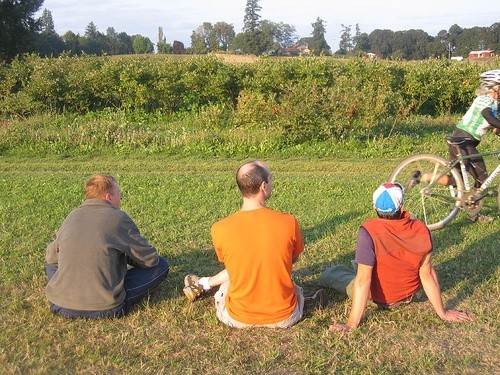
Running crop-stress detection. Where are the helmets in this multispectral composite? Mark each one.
[480,69,500,86]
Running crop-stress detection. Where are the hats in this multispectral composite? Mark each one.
[373,182,405,214]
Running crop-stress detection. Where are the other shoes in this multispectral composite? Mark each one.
[405,171,422,193]
[466,213,494,223]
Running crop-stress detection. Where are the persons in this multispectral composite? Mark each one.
[44,171,170,321]
[182,160,328,330]
[318,182,474,333]
[403,68,500,224]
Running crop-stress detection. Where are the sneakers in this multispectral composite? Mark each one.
[303,288,328,314]
[183,274,205,302]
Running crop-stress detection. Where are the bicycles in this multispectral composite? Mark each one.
[385,135,500,232]
